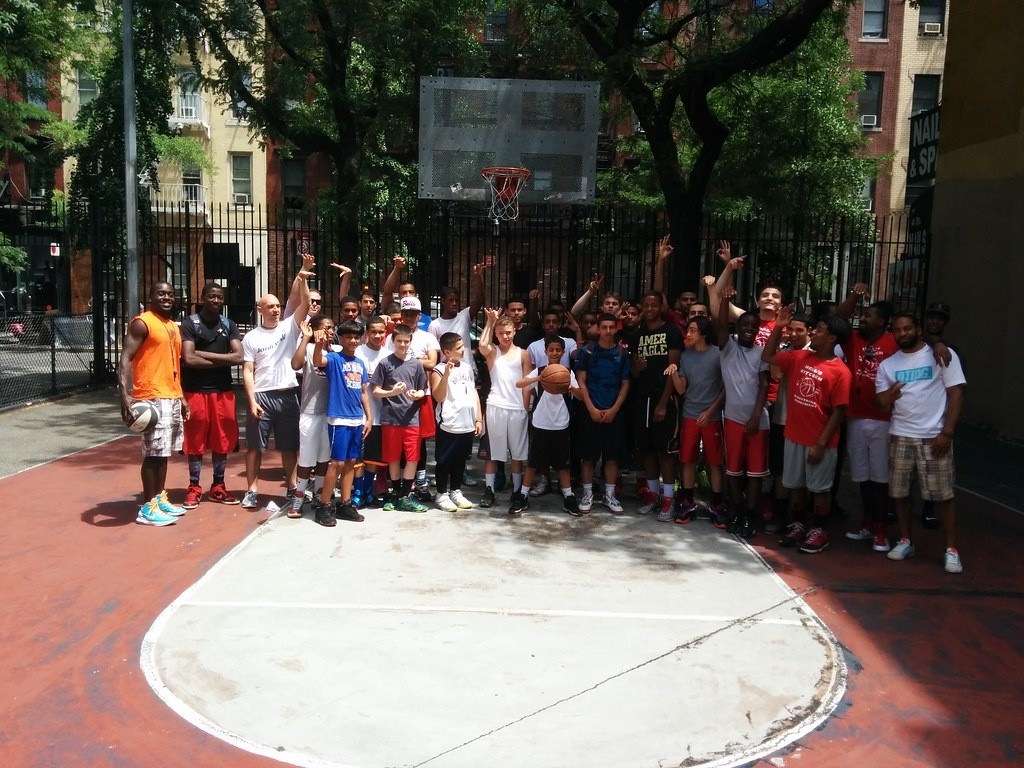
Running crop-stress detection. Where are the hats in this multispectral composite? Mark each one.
[401,296,421,311]
[926,301,953,316]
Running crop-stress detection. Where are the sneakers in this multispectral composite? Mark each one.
[426,464,896,554]
[944,551,962,573]
[135,496,179,528]
[336,501,364,522]
[397,493,429,513]
[156,490,186,517]
[311,488,332,508]
[352,494,366,507]
[314,504,337,526]
[242,490,258,508]
[285,487,312,503]
[287,490,305,518]
[434,491,456,512]
[208,482,240,505]
[367,493,383,507]
[183,483,202,508]
[382,491,401,510]
[450,489,473,509]
[886,537,915,560]
[412,487,431,502]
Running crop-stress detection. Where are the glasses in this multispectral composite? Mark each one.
[311,299,322,305]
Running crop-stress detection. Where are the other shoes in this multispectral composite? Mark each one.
[924,514,941,529]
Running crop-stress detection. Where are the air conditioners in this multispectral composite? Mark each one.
[181,107,196,117]
[861,200,872,212]
[861,115,878,126]
[912,109,928,116]
[923,22,941,34]
[234,193,249,205]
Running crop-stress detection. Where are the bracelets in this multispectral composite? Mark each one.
[475,420,483,423]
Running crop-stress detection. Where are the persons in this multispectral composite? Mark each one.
[478,272,685,521]
[178,283,243,508]
[241,254,486,524]
[656,232,967,573]
[117,282,186,526]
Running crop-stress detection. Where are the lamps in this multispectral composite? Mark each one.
[257,256,261,267]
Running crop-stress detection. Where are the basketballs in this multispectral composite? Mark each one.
[540,363,571,394]
